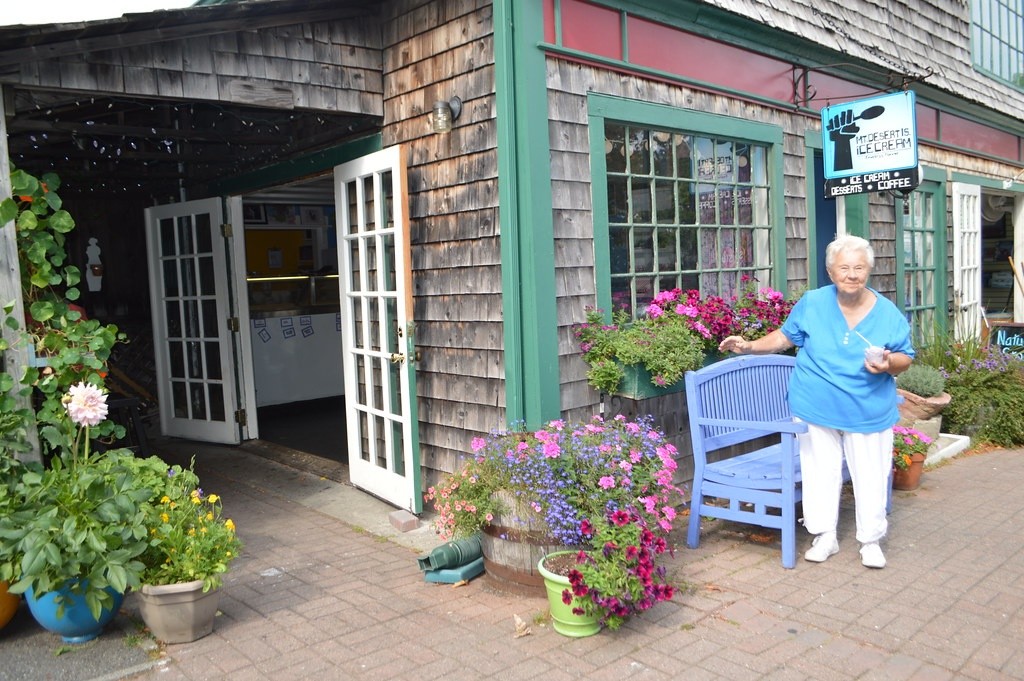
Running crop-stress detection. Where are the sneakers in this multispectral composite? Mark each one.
[859,542,886,568]
[805,534,840,562]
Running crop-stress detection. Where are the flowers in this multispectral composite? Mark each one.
[572,273,797,395]
[417,413,690,546]
[893,448,912,471]
[892,425,933,455]
[0,381,154,623]
[561,510,687,630]
[132,455,240,600]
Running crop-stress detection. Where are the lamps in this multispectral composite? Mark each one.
[878,165,924,215]
[1003,169,1024,189]
[604,130,747,173]
[431,95,462,134]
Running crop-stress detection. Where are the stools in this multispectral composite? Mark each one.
[90,392,152,459]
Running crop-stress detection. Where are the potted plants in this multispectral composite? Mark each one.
[893,364,951,421]
[1,413,33,627]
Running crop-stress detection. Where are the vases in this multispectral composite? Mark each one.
[138,580,221,644]
[603,342,796,401]
[538,550,609,638]
[892,452,927,490]
[480,483,593,599]
[9,560,154,646]
[893,465,897,483]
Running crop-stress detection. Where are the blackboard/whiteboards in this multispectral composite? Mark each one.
[986,321,1024,361]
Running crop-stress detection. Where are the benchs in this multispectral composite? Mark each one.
[684,355,904,569]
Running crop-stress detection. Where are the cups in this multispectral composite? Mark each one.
[865,347,884,366]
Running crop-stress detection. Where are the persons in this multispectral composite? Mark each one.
[718,236,916,568]
